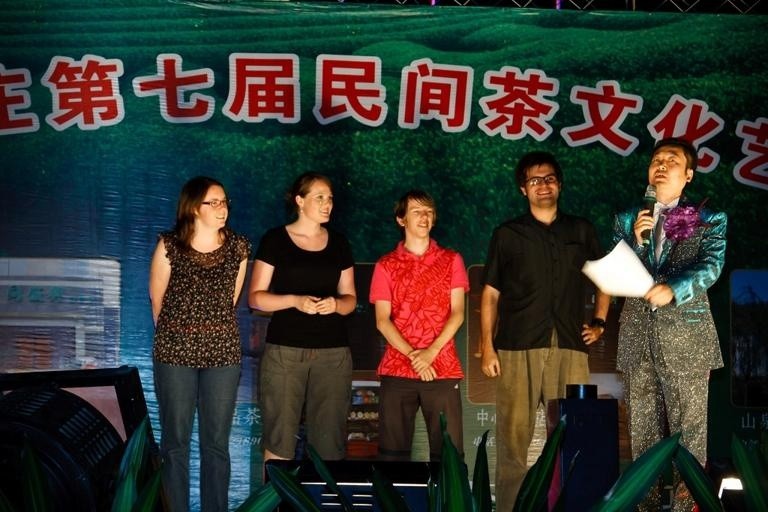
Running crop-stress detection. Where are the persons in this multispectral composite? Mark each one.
[480,150,612,512]
[247,171,359,488]
[145,175,256,510]
[608,136,731,511]
[368,188,472,467]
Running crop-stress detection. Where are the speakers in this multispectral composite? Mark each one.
[558,397,621,512]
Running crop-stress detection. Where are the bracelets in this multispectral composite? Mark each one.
[591,318,606,329]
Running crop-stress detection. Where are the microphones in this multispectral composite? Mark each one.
[640,184,658,246]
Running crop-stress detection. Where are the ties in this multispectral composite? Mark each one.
[653,209,665,251]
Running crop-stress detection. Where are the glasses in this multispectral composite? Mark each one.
[199,198,232,208]
[524,174,557,186]
